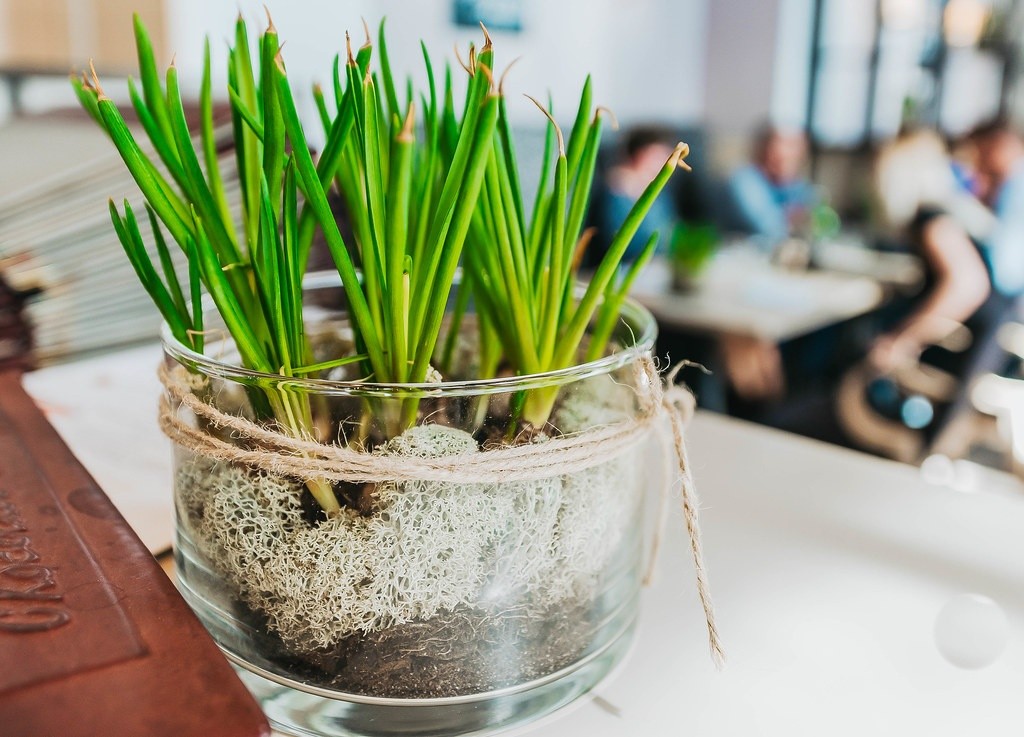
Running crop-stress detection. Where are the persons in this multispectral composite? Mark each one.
[601,121,786,395]
[835,122,1024,461]
[713,116,822,235]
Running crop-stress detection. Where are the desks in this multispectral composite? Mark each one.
[617,256,883,344]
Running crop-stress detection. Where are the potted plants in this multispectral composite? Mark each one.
[67,6,690,737]
